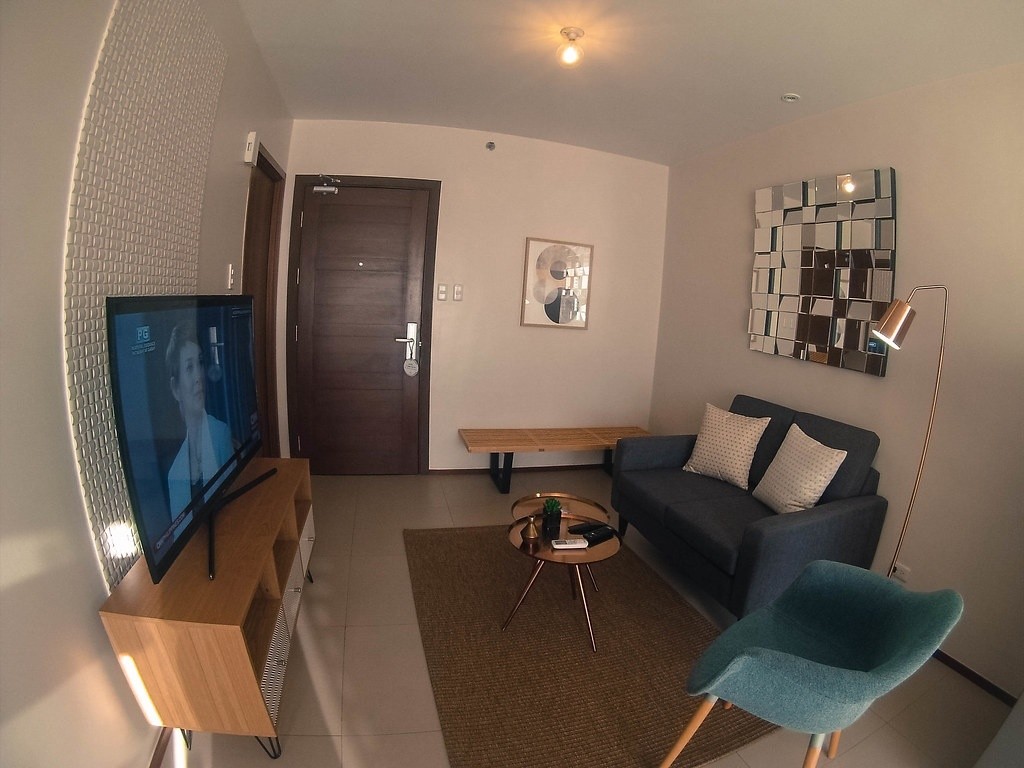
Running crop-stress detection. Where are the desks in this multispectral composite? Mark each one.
[502,492,623,653]
[458,427,656,496]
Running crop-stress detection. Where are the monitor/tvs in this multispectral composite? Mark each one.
[104,294,276,586]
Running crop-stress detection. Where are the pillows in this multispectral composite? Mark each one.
[683,402,772,492]
[752,423,848,516]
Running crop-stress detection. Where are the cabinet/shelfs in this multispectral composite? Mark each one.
[98,456,317,758]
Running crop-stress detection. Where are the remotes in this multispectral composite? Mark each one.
[583,525,614,541]
[552,538,588,550]
[568,522,606,535]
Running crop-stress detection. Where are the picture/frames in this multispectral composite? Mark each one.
[519,237,595,331]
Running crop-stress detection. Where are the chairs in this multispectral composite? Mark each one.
[646,559,964,768]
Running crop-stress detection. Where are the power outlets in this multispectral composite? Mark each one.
[892,562,911,582]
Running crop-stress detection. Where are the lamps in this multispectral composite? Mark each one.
[871,285,950,580]
[555,26,586,71]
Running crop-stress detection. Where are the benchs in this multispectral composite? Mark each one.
[610,394,888,628]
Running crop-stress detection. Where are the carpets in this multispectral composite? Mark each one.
[404,522,782,768]
